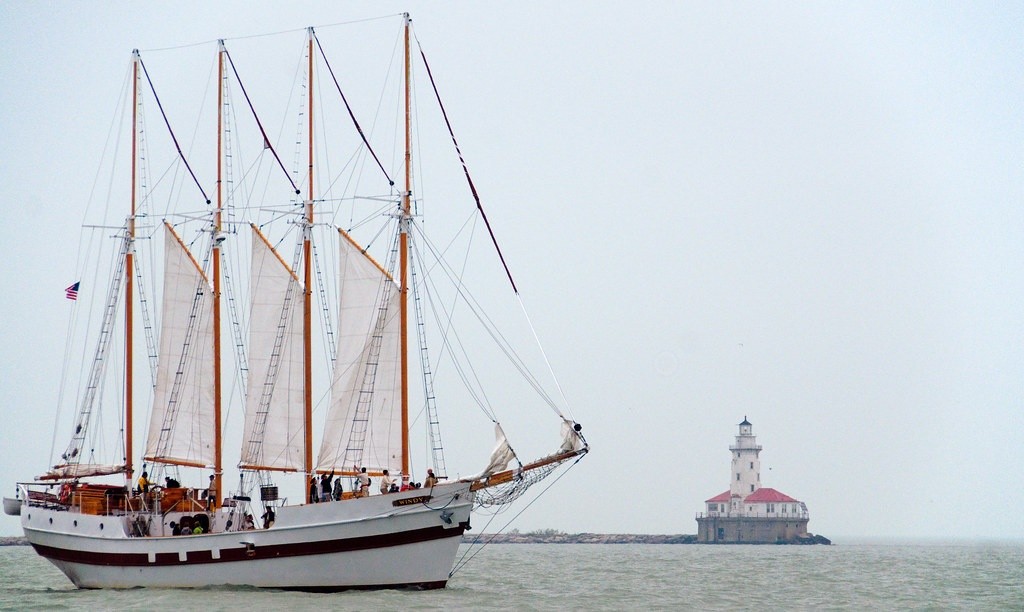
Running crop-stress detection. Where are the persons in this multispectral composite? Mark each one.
[380,470,399,494]
[165,477,180,488]
[261,506,274,529]
[193,521,203,533]
[409,482,421,490]
[137,472,148,495]
[242,512,254,529]
[424,470,436,488]
[353,465,369,497]
[181,522,192,535]
[206,475,216,510]
[310,471,342,504]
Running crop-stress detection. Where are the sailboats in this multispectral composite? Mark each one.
[1,11,590,590]
